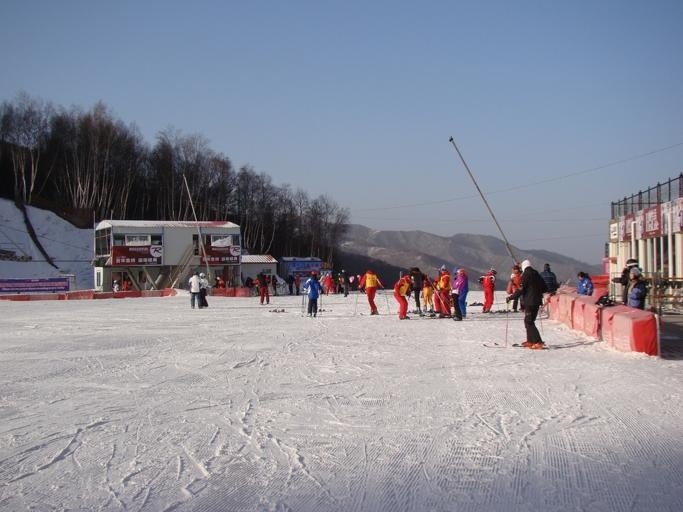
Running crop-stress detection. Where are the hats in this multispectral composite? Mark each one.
[631,268,640,276]
[514,265,519,271]
[441,264,464,275]
[311,270,319,275]
[489,268,497,274]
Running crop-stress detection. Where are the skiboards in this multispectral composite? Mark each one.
[483,342,575,350]
[538,278,571,316]
[265,308,335,318]
[360,301,522,321]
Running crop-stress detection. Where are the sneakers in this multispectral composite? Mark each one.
[260,302,269,305]
[521,341,544,349]
[370,311,379,315]
[414,308,467,321]
[191,303,209,309]
[307,313,316,317]
[482,308,493,313]
[399,315,410,320]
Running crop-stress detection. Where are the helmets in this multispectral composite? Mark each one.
[199,273,206,279]
[626,258,639,270]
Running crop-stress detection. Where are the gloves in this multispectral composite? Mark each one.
[506,297,510,303]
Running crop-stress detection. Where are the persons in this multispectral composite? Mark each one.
[111,279,120,294]
[188,269,384,317]
[507,265,525,313]
[539,263,557,293]
[122,278,129,291]
[575,272,593,296]
[505,260,547,350]
[611,259,642,305]
[177,270,185,289]
[627,267,645,309]
[392,263,468,321]
[477,269,497,313]
